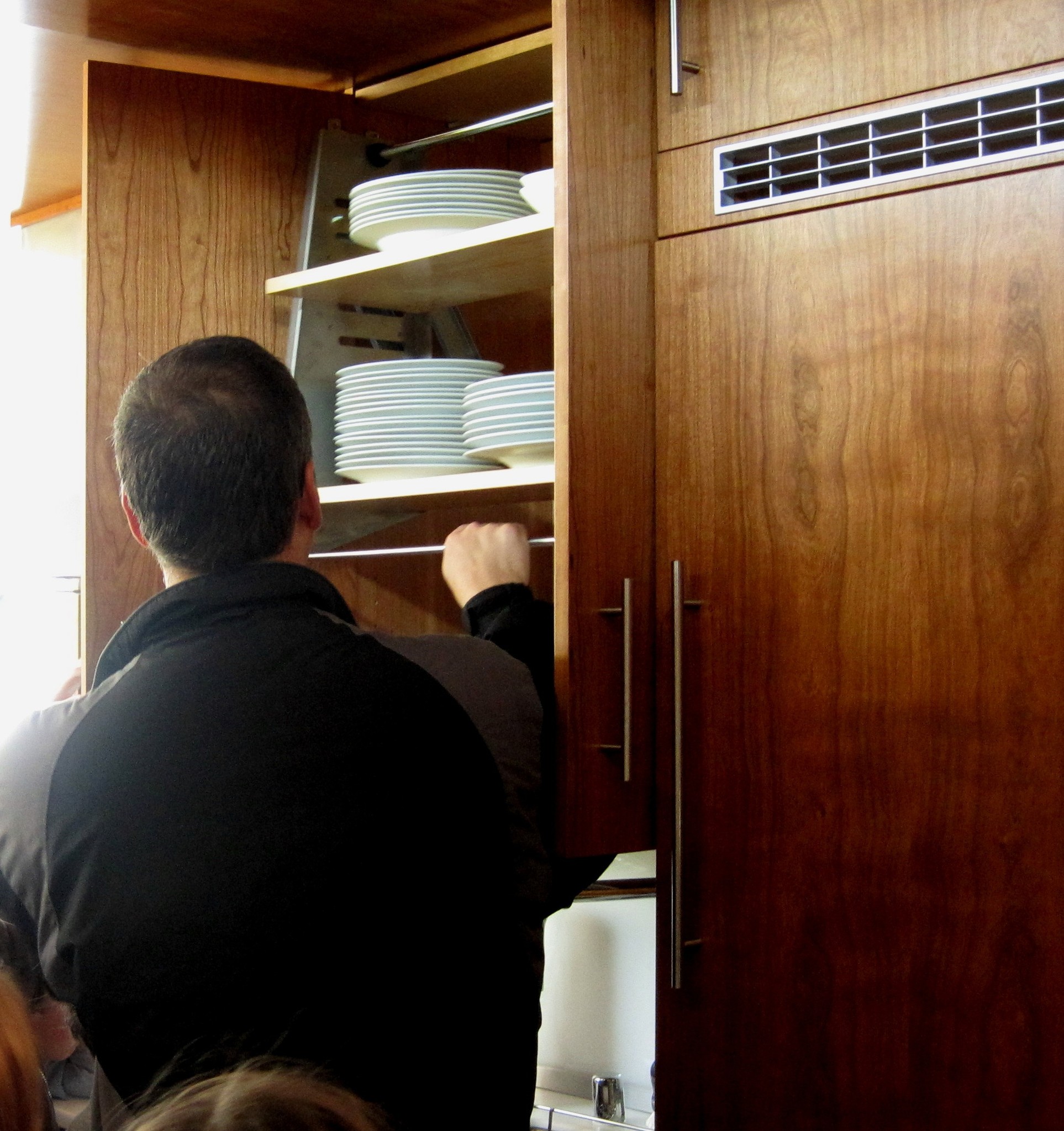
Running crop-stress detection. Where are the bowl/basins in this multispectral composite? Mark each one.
[519,168,553,214]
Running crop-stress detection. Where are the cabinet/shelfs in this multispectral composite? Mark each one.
[654,0,1064,154]
[656,163,1063,1131]
[265,96,553,505]
[74,0,654,855]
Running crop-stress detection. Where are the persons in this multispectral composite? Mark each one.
[0,333,579,1131]
[111,1066,372,1129]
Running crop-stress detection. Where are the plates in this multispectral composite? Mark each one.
[347,169,536,252]
[334,356,555,486]
